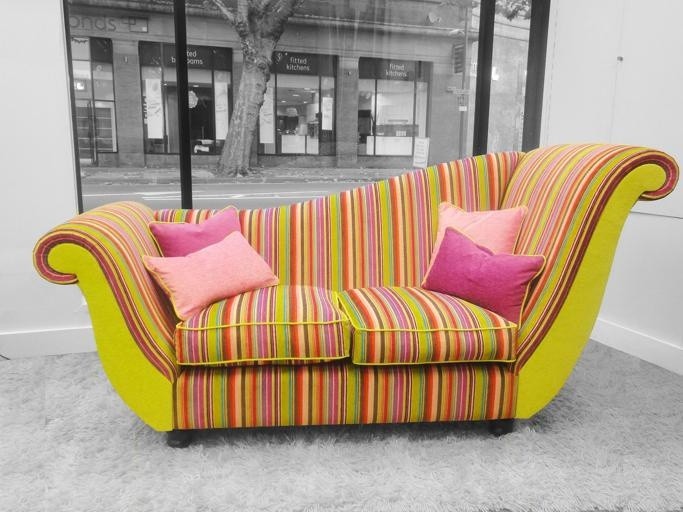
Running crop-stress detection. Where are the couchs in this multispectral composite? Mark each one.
[31,142,679,449]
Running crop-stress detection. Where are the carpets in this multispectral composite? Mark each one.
[0,338,682,510]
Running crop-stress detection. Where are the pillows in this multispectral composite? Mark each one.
[139,204,281,322]
[419,200,547,330]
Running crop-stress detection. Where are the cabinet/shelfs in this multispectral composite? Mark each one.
[73,100,117,152]
